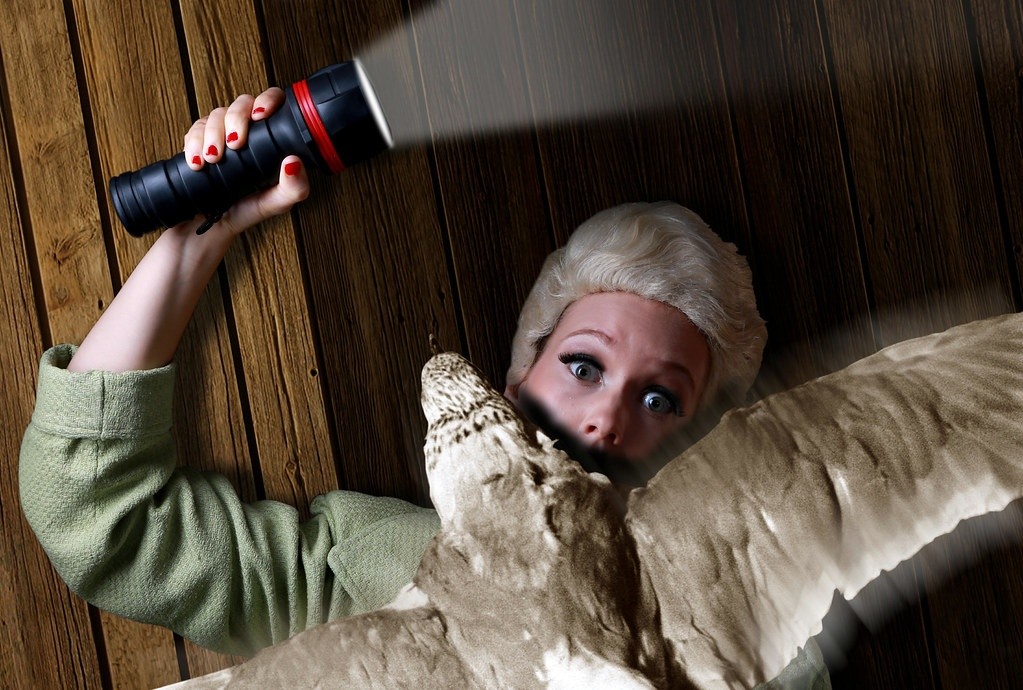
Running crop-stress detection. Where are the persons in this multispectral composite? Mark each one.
[18,58,831,690]
